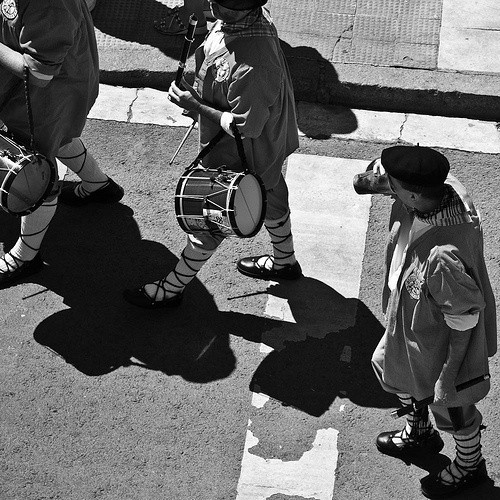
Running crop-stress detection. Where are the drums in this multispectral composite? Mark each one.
[174,167,267,238]
[0,129,55,214]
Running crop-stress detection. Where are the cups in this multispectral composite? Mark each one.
[353,170,397,195]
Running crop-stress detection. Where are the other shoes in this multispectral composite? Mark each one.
[420,459,494,499]
[122,286,186,308]
[238,256,304,281]
[0,251,41,287]
[375,429,444,454]
[57,179,125,212]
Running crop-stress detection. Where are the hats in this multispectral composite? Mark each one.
[215,0,268,11]
[380,142,451,184]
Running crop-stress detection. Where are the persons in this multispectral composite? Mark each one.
[0,0,302,309]
[365,143,497,500]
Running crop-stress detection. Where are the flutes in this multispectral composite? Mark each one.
[174,14,198,91]
